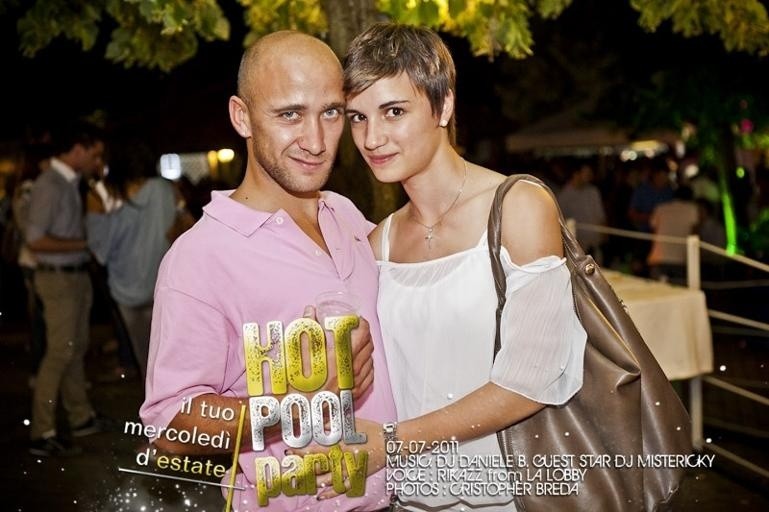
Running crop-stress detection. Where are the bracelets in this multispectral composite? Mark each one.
[382,422,404,467]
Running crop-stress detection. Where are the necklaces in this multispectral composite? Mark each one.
[408,155,469,250]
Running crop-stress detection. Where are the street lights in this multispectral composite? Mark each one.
[490,170,691,512]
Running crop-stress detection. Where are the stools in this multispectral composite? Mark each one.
[40,264,90,274]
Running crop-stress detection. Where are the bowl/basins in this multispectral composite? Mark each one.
[408,155,469,250]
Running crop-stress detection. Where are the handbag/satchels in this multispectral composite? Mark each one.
[382,422,404,467]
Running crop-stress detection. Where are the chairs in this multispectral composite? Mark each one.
[29,432,81,457]
[68,415,114,437]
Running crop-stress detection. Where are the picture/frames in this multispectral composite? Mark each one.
[587,266,720,387]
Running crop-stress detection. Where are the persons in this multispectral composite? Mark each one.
[343,23,588,512]
[138,28,405,512]
[0,115,218,458]
[553,148,769,290]
